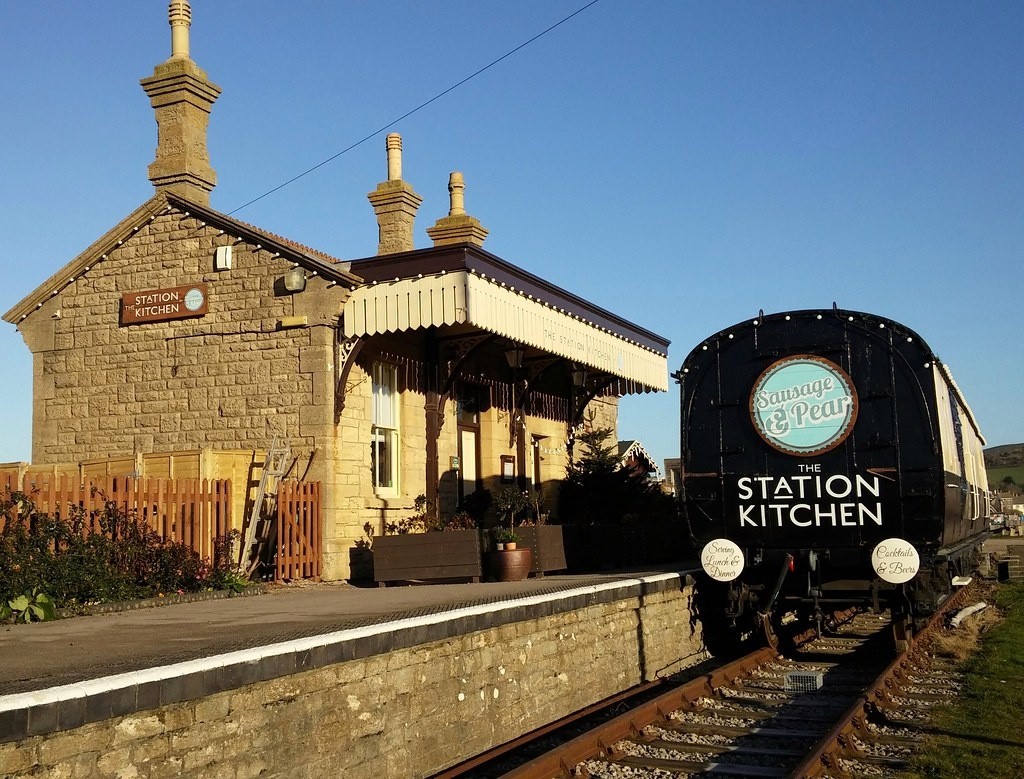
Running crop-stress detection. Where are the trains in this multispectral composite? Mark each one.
[670,301,994,651]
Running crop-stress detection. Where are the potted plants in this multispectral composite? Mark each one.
[374,494,490,587]
[489,485,535,582]
[506,489,604,579]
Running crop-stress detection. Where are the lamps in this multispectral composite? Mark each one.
[570,363,590,388]
[504,341,524,369]
